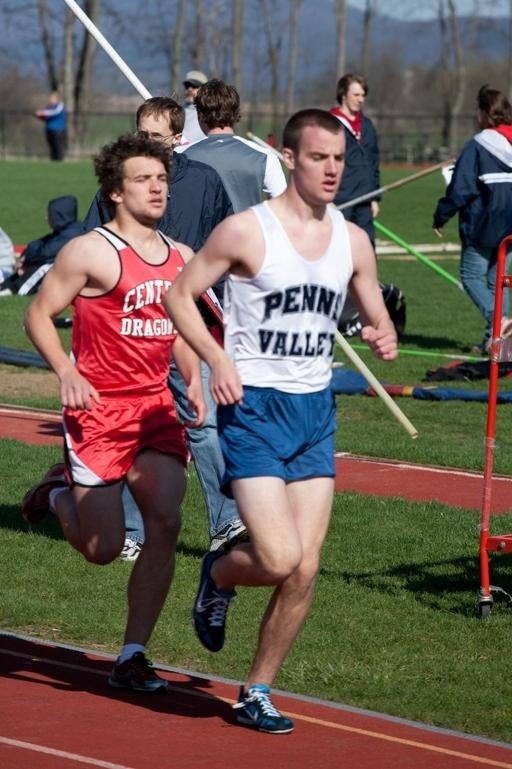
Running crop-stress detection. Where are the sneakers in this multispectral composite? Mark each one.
[18,462,72,524]
[208,519,250,556]
[119,537,144,563]
[234,684,296,736]
[106,648,171,693]
[192,549,239,653]
[482,317,512,356]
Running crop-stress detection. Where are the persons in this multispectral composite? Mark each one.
[34,90,67,161]
[181,71,208,135]
[85,98,249,559]
[159,108,397,733]
[328,72,381,292]
[434,84,512,343]
[3,195,85,293]
[22,132,207,696]
[0,225,14,287]
[182,79,287,211]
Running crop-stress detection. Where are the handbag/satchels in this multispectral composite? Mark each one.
[334,279,414,337]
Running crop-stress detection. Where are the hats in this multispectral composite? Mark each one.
[182,70,209,90]
